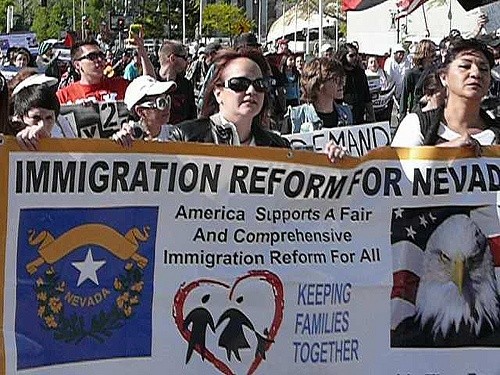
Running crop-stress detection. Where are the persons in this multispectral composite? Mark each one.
[0,13,500,163]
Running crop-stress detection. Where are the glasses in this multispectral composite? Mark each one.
[215,76,276,92]
[137,94,172,110]
[326,74,347,86]
[167,53,188,61]
[348,53,358,57]
[74,51,105,61]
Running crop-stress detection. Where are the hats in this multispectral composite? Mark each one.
[197,46,206,57]
[11,73,59,97]
[393,44,406,54]
[278,39,290,44]
[450,29,461,37]
[321,43,333,51]
[124,75,178,111]
[239,33,262,47]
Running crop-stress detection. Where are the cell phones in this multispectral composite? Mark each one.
[128,24,142,48]
